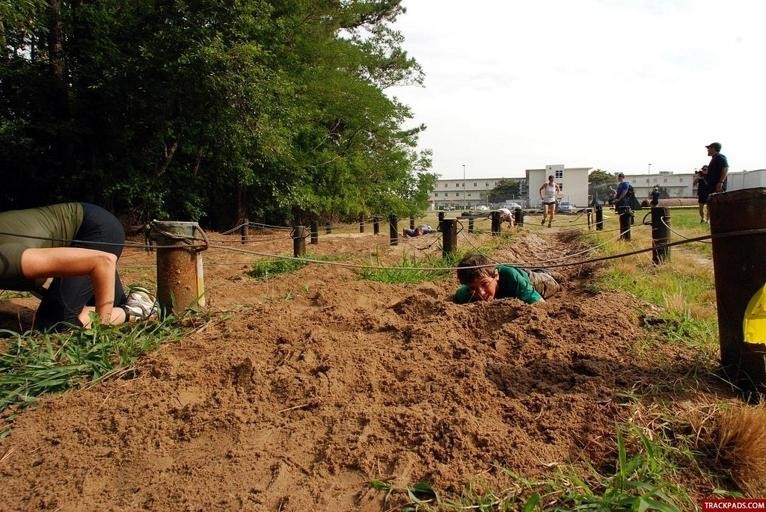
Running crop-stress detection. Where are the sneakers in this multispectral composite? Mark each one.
[121,286,158,320]
[700,219,710,224]
[541,220,551,227]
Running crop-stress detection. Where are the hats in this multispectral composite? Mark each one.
[705,142,721,151]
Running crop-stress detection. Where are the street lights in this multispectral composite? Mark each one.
[648,163,652,199]
[462,164,466,209]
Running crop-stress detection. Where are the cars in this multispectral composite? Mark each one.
[471,202,521,210]
[444,206,456,211]
[558,201,572,212]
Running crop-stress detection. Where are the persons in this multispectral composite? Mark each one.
[402,225,438,238]
[452,255,562,306]
[607,174,633,226]
[649,188,660,207]
[540,175,560,227]
[0,202,159,332]
[694,143,727,224]
[495,208,515,230]
